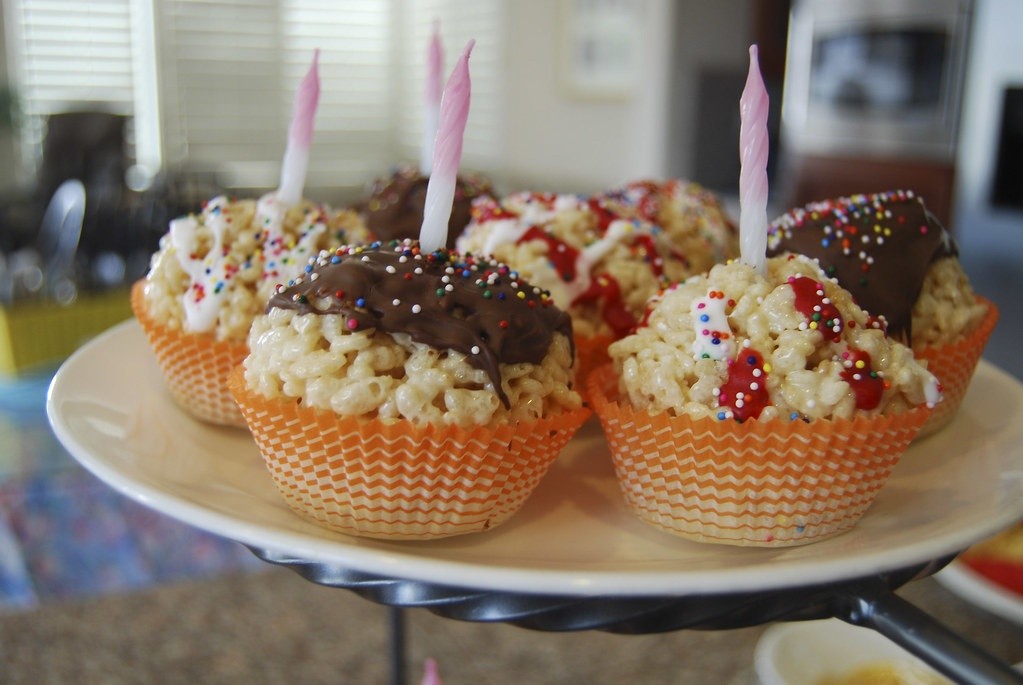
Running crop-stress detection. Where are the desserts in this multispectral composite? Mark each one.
[129,166,999,550]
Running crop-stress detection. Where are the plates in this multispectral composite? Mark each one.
[754,620,955,685]
[932,554,1023,626]
[47,317,1023,596]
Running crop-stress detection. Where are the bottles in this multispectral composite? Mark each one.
[776,0,975,235]
